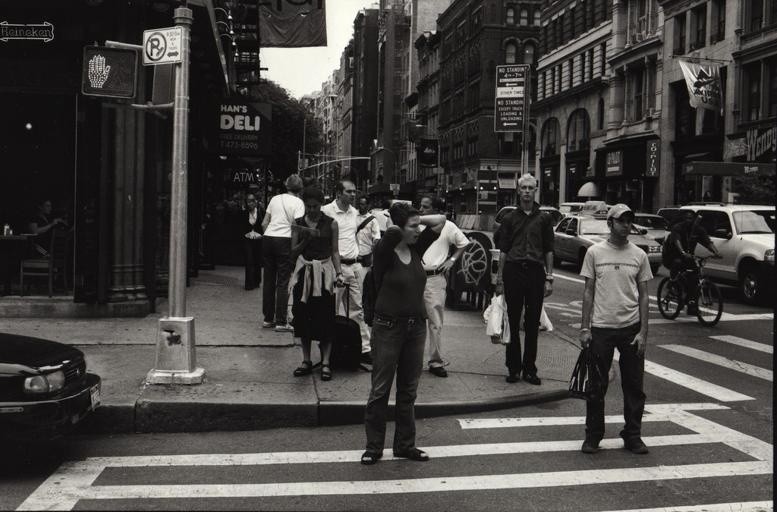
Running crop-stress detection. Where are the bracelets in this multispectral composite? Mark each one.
[497,282,504,286]
[580,328,591,333]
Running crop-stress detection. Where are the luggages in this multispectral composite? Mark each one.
[317,282,362,372]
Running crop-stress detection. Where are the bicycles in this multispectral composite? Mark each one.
[658,253,723,326]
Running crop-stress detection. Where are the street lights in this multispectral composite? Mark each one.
[303,94,339,177]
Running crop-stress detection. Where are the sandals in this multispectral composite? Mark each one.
[293,360,312,376]
[361,451,383,464]
[321,364,332,380]
[395,448,428,461]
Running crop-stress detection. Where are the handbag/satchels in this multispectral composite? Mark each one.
[569,347,609,403]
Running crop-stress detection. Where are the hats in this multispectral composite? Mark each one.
[607,204,633,220]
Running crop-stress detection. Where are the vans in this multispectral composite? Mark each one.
[495,207,562,231]
[634,213,671,244]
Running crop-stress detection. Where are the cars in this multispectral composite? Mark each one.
[554,202,663,273]
[0,333,101,445]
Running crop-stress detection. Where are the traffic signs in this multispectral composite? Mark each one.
[142,26,184,65]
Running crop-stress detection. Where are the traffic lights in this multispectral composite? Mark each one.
[81,47,139,97]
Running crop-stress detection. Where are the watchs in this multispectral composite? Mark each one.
[545,279,553,283]
[680,250,685,255]
[336,272,344,278]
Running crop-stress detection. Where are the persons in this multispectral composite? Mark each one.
[492,173,554,386]
[579,204,655,455]
[417,193,470,378]
[369,174,390,200]
[355,196,381,268]
[241,194,266,291]
[321,179,373,364]
[661,210,723,316]
[261,174,305,332]
[369,200,394,237]
[27,199,68,293]
[291,187,346,381]
[359,202,447,465]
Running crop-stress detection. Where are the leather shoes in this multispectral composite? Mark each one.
[523,372,541,385]
[582,436,603,453]
[506,372,521,383]
[275,323,294,332]
[624,438,649,454]
[429,364,447,377]
[264,321,275,328]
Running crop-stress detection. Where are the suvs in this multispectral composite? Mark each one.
[656,206,777,304]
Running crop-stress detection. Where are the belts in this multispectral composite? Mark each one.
[514,261,539,270]
[425,270,441,275]
[340,257,358,264]
